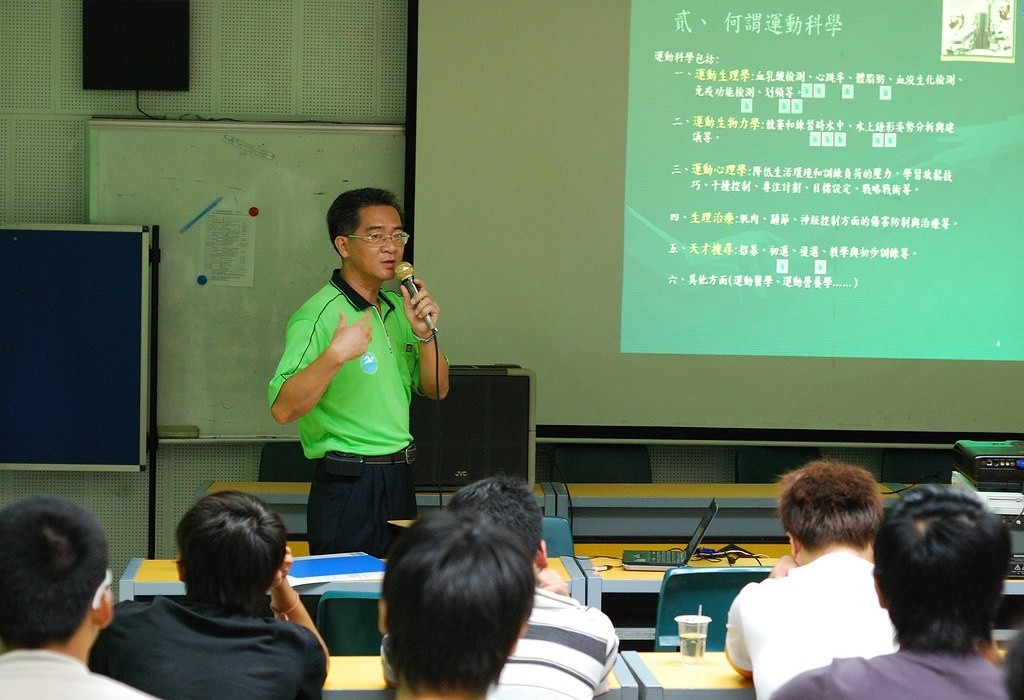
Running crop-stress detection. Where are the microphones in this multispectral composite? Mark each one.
[395,262,436,331]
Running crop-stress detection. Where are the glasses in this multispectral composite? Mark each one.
[339,231,410,247]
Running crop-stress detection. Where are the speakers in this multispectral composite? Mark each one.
[83,0,188,92]
[410,363,535,496]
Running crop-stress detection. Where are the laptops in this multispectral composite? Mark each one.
[623,498,719,571]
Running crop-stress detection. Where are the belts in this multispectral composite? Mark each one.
[325,444,418,464]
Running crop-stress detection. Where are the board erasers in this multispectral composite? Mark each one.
[158,425,199,439]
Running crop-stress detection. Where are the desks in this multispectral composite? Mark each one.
[550,481,1024,555]
[321,653,640,700]
[618,650,757,700]
[574,556,1024,642]
[119,557,587,608]
[194,478,556,535]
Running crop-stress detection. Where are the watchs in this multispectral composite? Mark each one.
[411,329,437,344]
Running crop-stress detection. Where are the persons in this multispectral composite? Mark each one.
[1005,633,1024,700]
[0,497,162,700]
[377,510,535,700]
[268,187,450,559]
[89,489,330,700]
[769,484,1021,700]
[724,459,903,700]
[446,473,619,700]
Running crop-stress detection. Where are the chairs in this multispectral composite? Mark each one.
[654,567,773,651]
[259,441,316,482]
[314,591,385,657]
[550,443,655,544]
[735,446,823,483]
[539,515,578,557]
[881,452,953,481]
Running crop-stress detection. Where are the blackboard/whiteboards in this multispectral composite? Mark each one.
[82,118,406,444]
[0,221,151,472]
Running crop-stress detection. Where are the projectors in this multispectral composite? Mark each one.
[951,440,1023,492]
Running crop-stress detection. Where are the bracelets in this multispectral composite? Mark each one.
[268,594,301,619]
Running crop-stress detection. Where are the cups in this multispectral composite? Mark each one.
[674,615,712,666]
